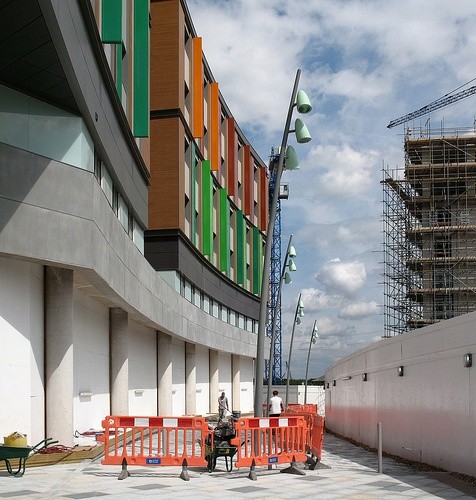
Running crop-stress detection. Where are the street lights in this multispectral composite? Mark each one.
[266,232,297,417]
[254,67,313,453]
[285,292,305,407]
[304,320,319,404]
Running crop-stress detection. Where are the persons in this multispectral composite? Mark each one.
[269,390,284,417]
[218,392,229,417]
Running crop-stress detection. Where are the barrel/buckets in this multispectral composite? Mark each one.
[4,433,28,448]
[232,410,241,422]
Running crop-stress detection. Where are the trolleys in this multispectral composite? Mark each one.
[196,438,250,473]
[0,438,59,477]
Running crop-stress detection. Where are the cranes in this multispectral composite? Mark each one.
[386,77,476,129]
